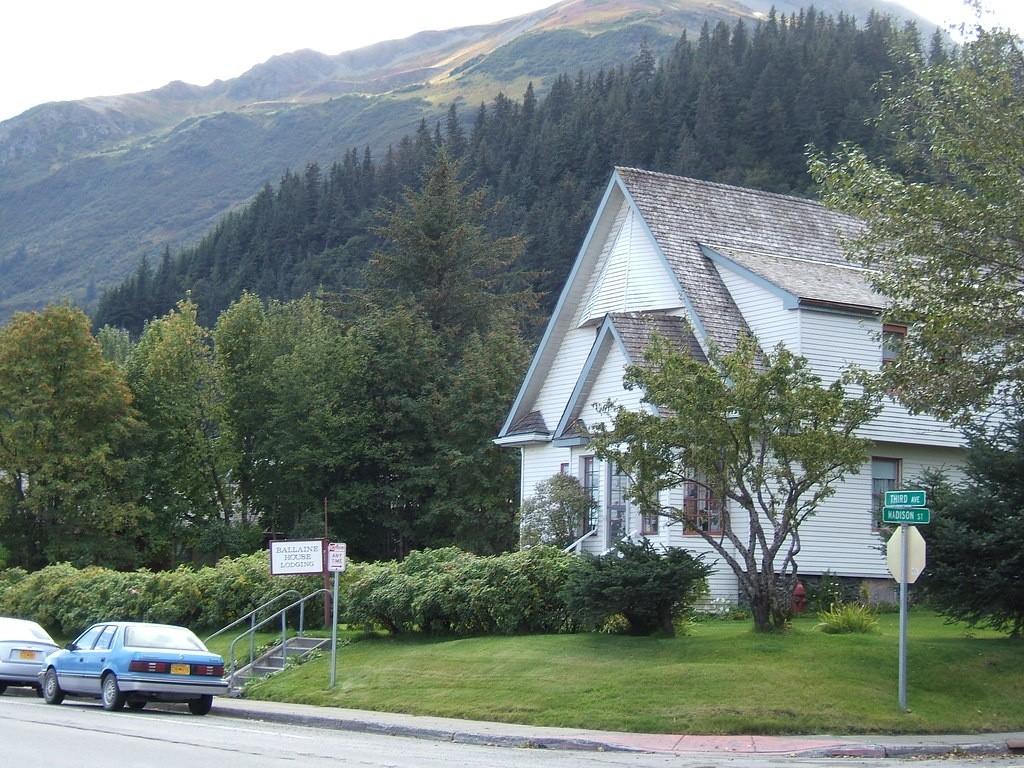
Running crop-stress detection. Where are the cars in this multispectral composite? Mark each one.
[37,621,230,715]
[0,616,62,697]
